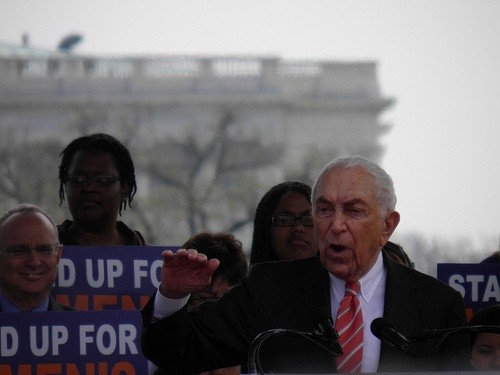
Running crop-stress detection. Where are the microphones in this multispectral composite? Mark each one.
[370,318,412,355]
[311,306,343,354]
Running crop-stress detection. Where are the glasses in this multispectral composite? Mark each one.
[267,212,314,228]
[64,174,121,191]
[0,243,61,262]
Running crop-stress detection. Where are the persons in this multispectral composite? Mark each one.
[52,134,152,247]
[153,231,248,375]
[139,157,470,375]
[468,249,500,373]
[249,181,324,267]
[0,204,78,312]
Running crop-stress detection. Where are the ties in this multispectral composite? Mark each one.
[332,279,364,375]
